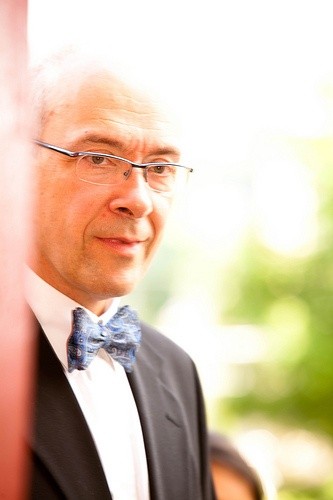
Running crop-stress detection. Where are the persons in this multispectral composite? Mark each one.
[21,52,216,500]
[205,427,264,500]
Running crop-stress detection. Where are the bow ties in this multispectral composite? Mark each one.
[65,304,141,371]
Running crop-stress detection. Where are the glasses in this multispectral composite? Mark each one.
[34,137,196,193]
